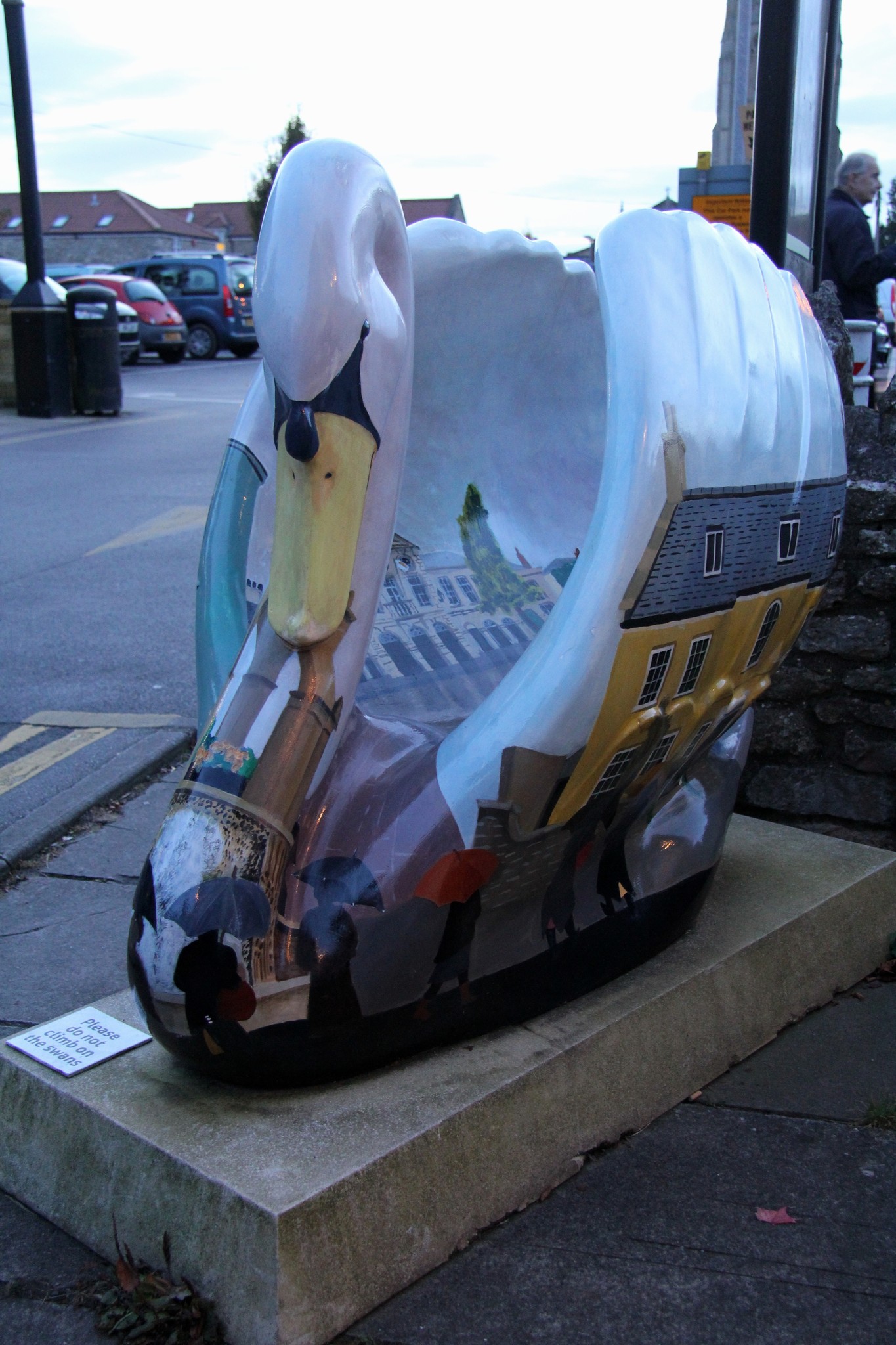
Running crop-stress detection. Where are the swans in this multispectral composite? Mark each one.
[131,140,846,1088]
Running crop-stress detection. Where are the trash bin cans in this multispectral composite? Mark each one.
[65,286,123,416]
[10,307,78,420]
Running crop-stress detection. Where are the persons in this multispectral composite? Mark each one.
[821,151,896,410]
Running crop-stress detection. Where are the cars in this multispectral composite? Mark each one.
[0,258,191,367]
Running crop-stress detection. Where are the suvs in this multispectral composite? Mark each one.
[107,252,261,361]
